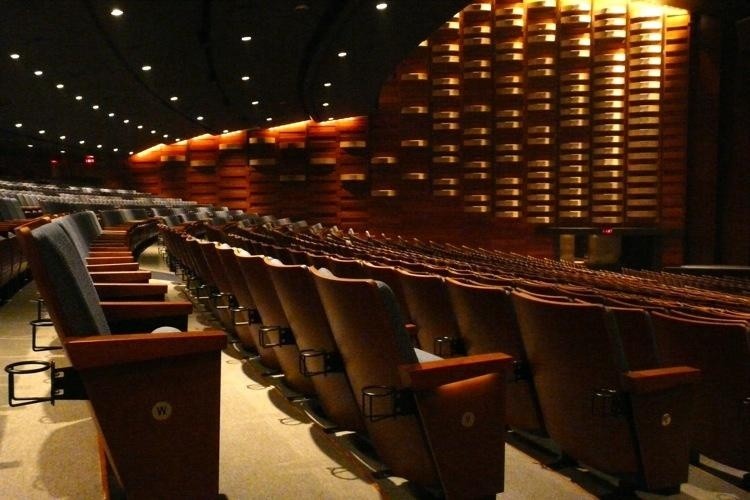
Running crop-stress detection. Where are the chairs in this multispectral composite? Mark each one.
[0,180,750,500]
